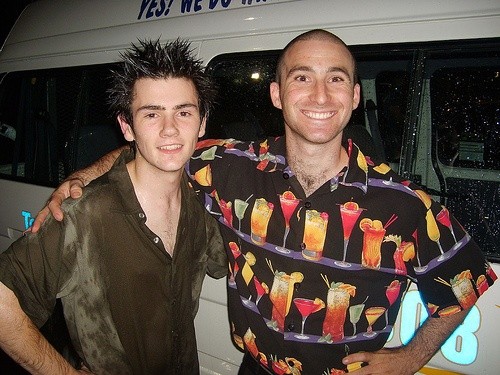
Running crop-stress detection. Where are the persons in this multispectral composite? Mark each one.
[23,29,497,375]
[0,35,233,375]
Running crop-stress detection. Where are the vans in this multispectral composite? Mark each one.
[0,0,500,375]
